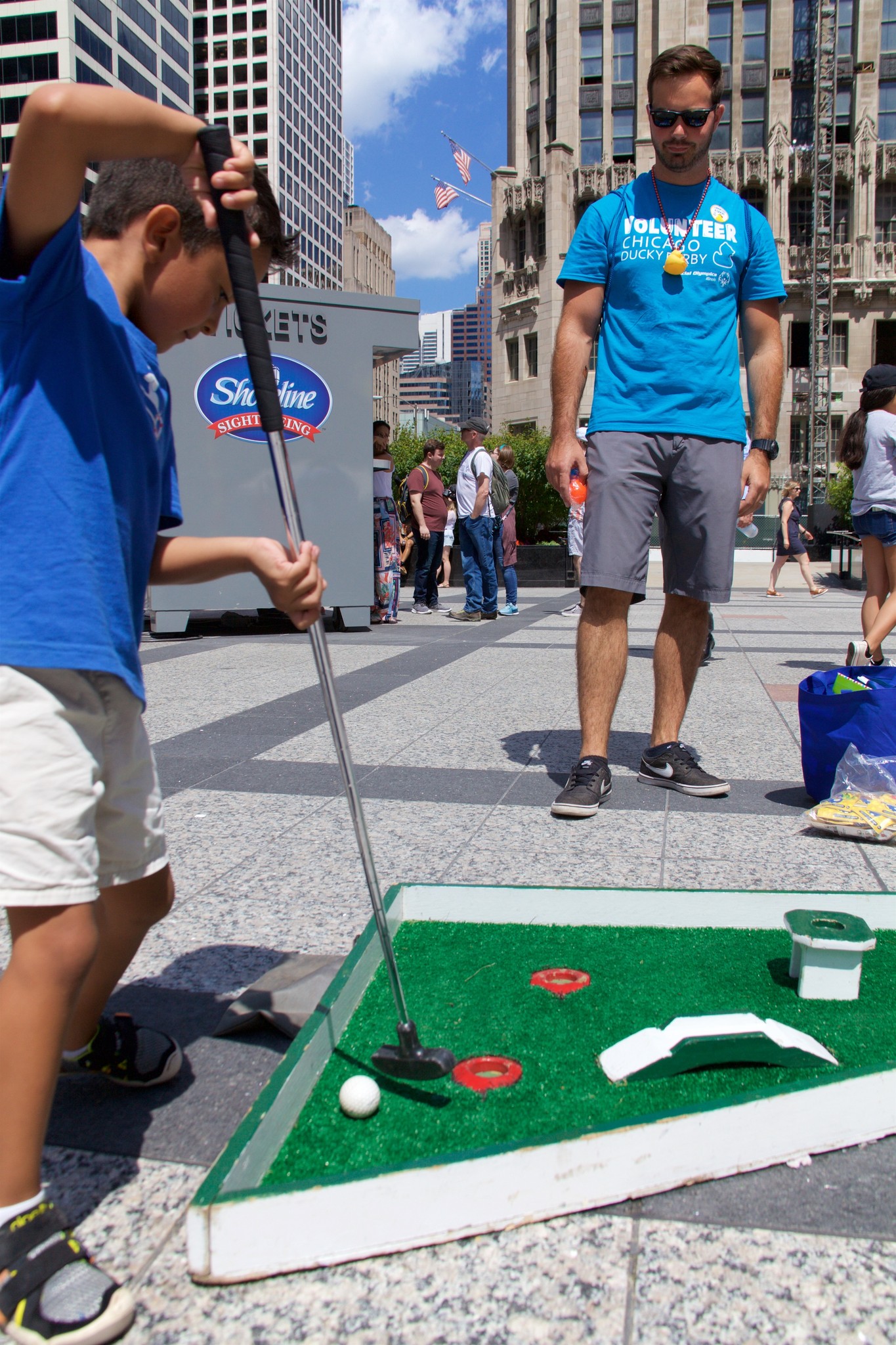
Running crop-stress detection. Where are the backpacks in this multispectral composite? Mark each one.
[462,448,510,516]
[396,465,443,525]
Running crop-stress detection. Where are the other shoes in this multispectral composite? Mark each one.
[400,566,407,575]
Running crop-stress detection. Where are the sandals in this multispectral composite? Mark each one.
[437,582,450,588]
[810,588,828,599]
[766,589,784,598]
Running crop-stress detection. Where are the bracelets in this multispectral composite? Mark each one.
[802,530,806,534]
[405,536,409,540]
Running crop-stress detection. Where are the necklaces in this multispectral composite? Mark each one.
[646,165,712,274]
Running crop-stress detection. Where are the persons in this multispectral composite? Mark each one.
[545,44,789,819]
[448,416,497,621]
[392,498,416,574]
[407,438,452,614]
[561,425,587,618]
[490,443,519,615]
[1,82,329,1345]
[836,363,895,667]
[434,484,457,588]
[370,420,402,624]
[765,480,829,599]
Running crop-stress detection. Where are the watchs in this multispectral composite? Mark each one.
[749,438,780,461]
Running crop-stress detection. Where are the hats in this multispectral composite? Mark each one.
[858,364,896,392]
[457,416,490,435]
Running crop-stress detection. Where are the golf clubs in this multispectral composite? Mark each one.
[185,126,461,1066]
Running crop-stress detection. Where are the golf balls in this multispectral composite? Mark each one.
[330,1074,382,1117]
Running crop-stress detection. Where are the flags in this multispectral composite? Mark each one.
[450,139,471,185]
[434,181,460,209]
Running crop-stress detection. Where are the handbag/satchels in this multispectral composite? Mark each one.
[492,502,516,540]
[798,666,896,804]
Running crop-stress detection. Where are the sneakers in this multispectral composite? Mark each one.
[428,603,452,613]
[0,1197,136,1345]
[449,608,481,622]
[868,656,896,667]
[638,743,731,797]
[845,640,873,666]
[56,1013,183,1090]
[479,607,498,620]
[411,600,432,614]
[497,602,519,616]
[550,758,613,816]
[561,604,584,617]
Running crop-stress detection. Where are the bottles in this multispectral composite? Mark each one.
[736,517,758,538]
[569,468,587,510]
[402,539,406,544]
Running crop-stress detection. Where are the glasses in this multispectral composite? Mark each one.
[460,428,473,433]
[792,488,801,492]
[491,452,500,455]
[647,104,715,128]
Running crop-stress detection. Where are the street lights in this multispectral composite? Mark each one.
[373,395,418,440]
